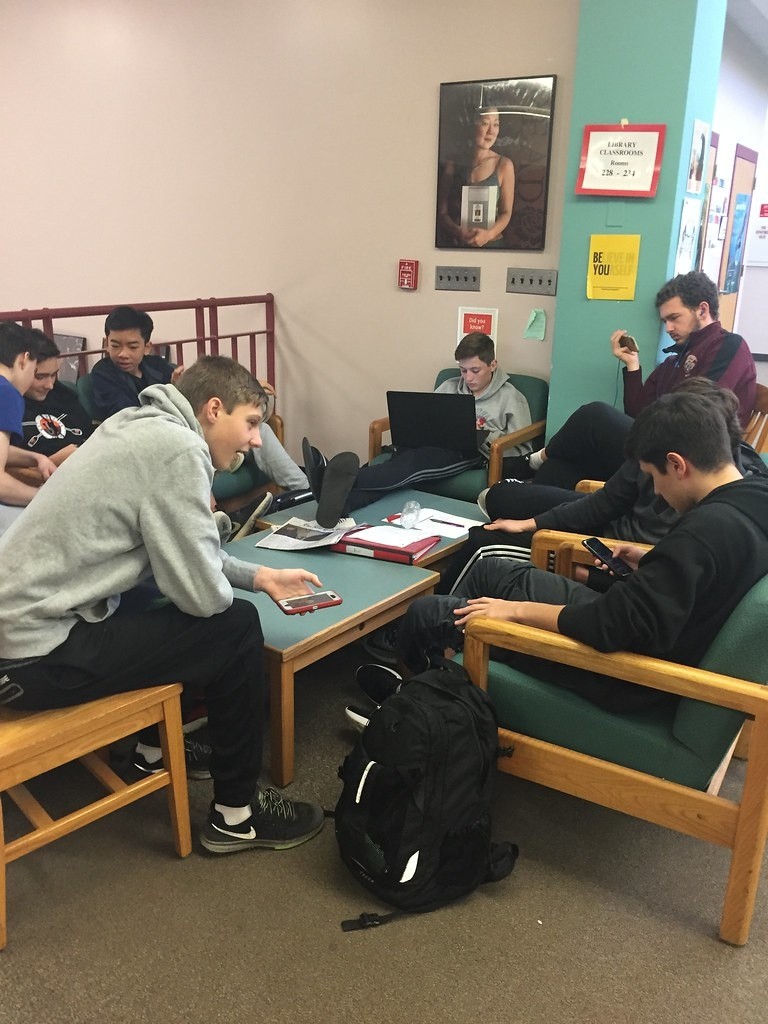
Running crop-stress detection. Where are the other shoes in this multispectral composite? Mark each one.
[477,487,492,520]
[229,451,244,473]
[301,436,331,504]
[488,452,536,479]
[317,451,361,529]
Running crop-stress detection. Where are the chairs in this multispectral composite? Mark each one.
[449,529,768,949]
[573,382,768,495]
[0,682,193,950]
[211,415,284,515]
[369,368,549,504]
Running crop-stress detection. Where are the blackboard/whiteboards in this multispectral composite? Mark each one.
[54,334,86,383]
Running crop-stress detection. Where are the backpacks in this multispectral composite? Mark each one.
[333,655,519,913]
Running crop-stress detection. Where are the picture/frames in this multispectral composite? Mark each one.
[434,74,558,253]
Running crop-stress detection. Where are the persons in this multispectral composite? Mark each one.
[520,269,758,489]
[301,332,534,528]
[0,319,274,545]
[443,105,515,249]
[345,392,768,727]
[362,376,766,664]
[0,354,325,856]
[91,302,311,493]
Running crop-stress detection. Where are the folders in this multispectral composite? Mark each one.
[330,524,441,565]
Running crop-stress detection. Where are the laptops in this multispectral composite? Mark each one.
[387,391,489,453]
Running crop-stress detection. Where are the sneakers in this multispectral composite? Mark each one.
[132,733,215,779]
[216,492,273,545]
[355,663,404,706]
[344,706,374,726]
[199,784,325,853]
[212,510,232,545]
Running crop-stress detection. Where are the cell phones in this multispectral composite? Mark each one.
[277,589,343,615]
[619,335,640,353]
[581,538,633,578]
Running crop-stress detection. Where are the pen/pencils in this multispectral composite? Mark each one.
[429,519,465,527]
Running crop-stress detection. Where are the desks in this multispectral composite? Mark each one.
[257,488,492,568]
[217,527,442,789]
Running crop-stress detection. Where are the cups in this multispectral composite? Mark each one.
[400,499,421,531]
